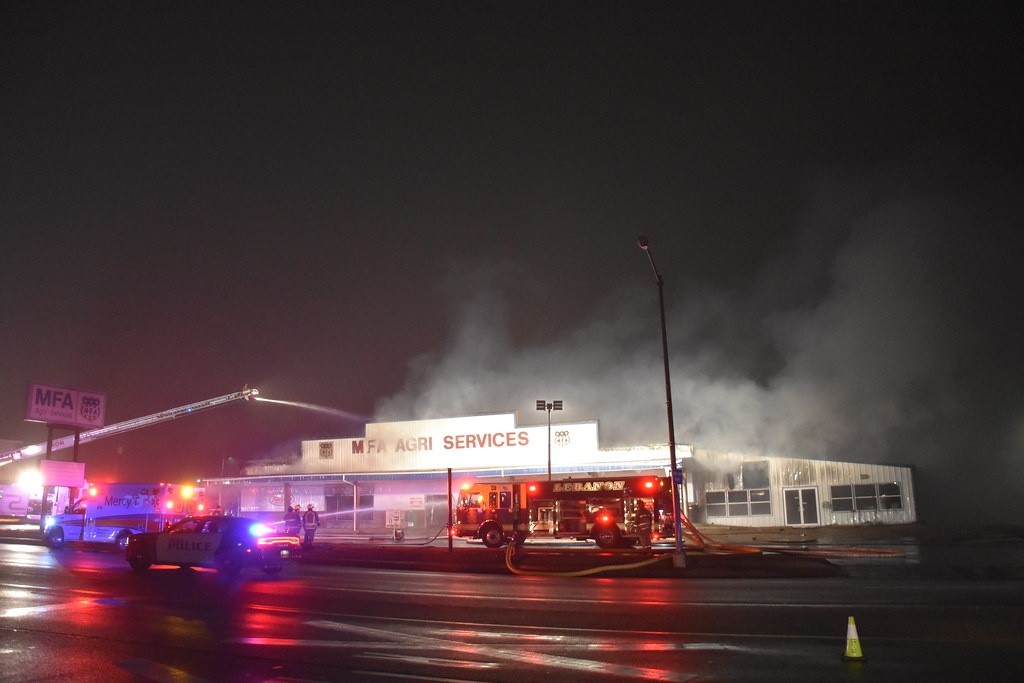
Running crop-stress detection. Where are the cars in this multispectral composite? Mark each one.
[123,515,303,579]
[27,499,51,516]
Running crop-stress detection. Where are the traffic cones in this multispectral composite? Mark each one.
[841,616,868,663]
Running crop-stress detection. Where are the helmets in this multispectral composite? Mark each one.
[307,504,314,509]
[294,505,301,510]
[287,505,294,511]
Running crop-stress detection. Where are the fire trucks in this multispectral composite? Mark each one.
[453,476,681,549]
[0,485,28,514]
[37,483,209,552]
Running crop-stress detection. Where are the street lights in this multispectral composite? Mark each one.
[638,234,690,569]
[536,400,562,481]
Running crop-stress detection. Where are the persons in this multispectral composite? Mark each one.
[633,500,652,556]
[212,505,222,517]
[284,504,321,548]
